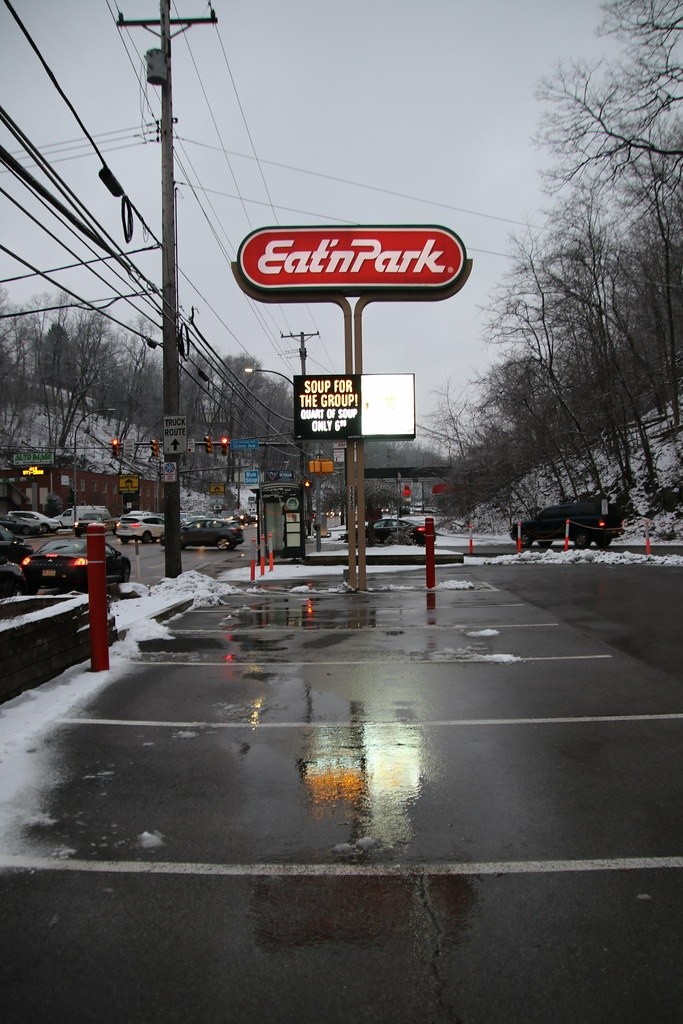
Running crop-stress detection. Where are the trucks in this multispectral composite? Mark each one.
[52,505,111,530]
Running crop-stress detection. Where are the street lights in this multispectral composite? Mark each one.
[73,408,115,526]
[244,367,312,537]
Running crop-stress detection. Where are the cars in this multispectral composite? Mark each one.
[111,511,166,535]
[22,537,131,596]
[180,518,245,551]
[116,515,166,543]
[0,525,34,566]
[0,515,39,536]
[343,517,436,546]
[180,512,258,527]
[0,555,26,599]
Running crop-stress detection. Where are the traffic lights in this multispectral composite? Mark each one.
[150,440,159,457]
[221,438,227,457]
[112,439,119,457]
[304,481,313,488]
[204,435,213,454]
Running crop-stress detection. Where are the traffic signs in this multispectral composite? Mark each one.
[163,415,187,454]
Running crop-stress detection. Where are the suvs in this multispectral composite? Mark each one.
[5,511,62,534]
[510,501,626,549]
[74,510,117,538]
